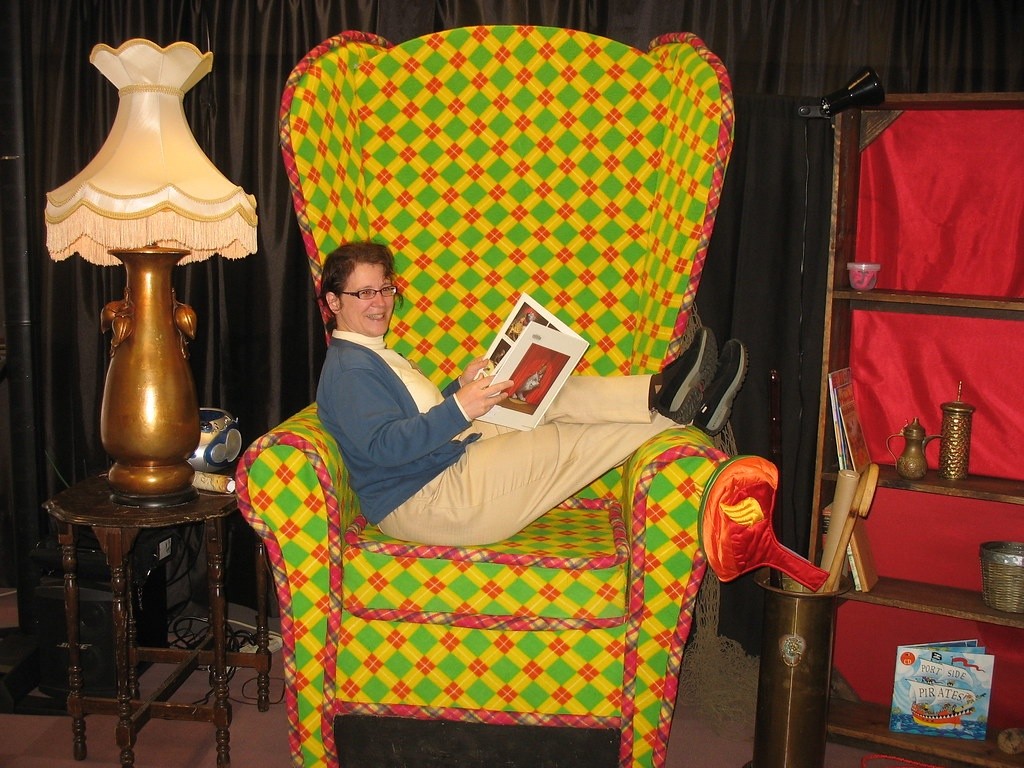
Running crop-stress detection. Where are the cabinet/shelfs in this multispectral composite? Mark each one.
[802,92,1024,768]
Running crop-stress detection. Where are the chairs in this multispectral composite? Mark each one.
[234,23,734,768]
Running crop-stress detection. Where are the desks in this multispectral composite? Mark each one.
[46,473,270,767]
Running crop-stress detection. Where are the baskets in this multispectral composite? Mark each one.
[979,540,1024,614]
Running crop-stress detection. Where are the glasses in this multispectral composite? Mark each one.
[333,285,397,300]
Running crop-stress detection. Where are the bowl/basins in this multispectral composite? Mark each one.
[846,263,880,290]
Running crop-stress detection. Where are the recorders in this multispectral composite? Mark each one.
[188,408,243,474]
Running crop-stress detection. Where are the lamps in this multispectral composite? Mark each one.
[819,64,887,117]
[44,38,260,505]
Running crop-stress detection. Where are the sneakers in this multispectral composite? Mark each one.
[652,328,719,424]
[692,338,749,437]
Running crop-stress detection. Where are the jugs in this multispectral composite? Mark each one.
[886,416,944,481]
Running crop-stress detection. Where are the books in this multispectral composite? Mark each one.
[829,367,874,474]
[470,292,590,434]
[887,639,997,741]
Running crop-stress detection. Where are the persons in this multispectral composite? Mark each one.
[314,242,750,547]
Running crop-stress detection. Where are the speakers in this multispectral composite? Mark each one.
[16,532,172,702]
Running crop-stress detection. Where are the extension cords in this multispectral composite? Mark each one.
[208,635,283,677]
